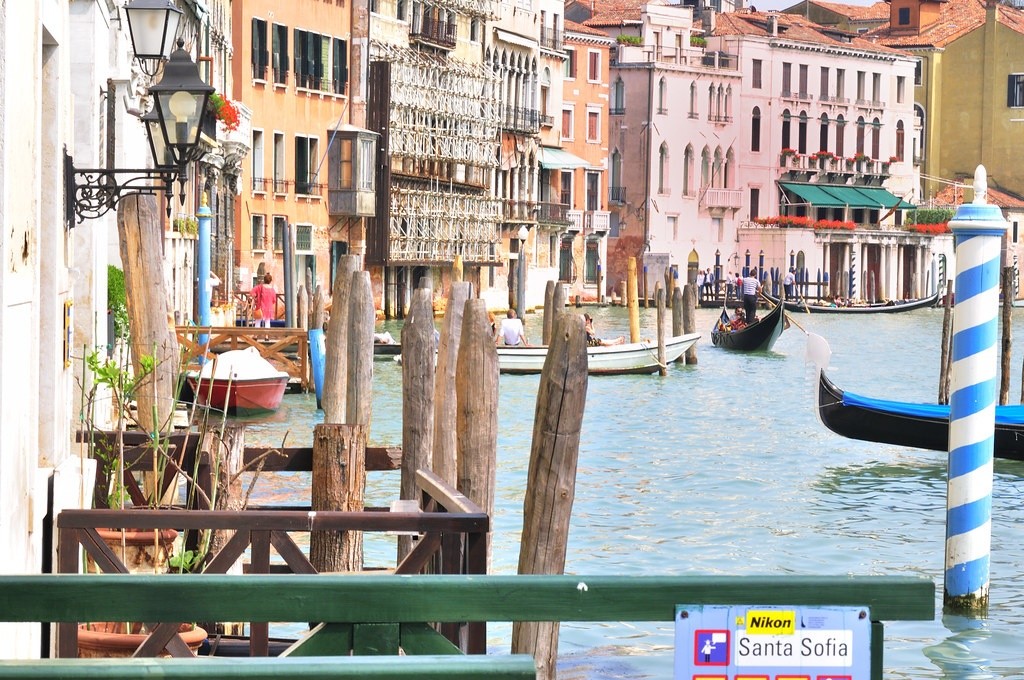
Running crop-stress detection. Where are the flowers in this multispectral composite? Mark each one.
[781,148,897,169]
[752,216,856,231]
[909,221,953,236]
[204,91,241,135]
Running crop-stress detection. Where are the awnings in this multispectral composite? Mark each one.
[536,146,590,170]
[776,180,917,211]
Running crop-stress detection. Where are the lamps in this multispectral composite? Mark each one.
[63,0,216,232]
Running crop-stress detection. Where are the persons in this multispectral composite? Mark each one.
[256,262,268,285]
[250,272,276,341]
[696,267,848,308]
[489,309,626,349]
[737,269,762,324]
[210,270,220,308]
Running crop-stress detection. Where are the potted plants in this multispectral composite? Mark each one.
[616,35,642,48]
[690,37,707,48]
[68,316,221,658]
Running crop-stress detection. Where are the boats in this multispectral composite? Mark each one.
[819,367,1024,462]
[434,334,702,375]
[187,350,290,417]
[711,293,785,352]
[763,289,939,314]
[374,331,403,358]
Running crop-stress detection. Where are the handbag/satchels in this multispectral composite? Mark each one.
[252,310,263,320]
